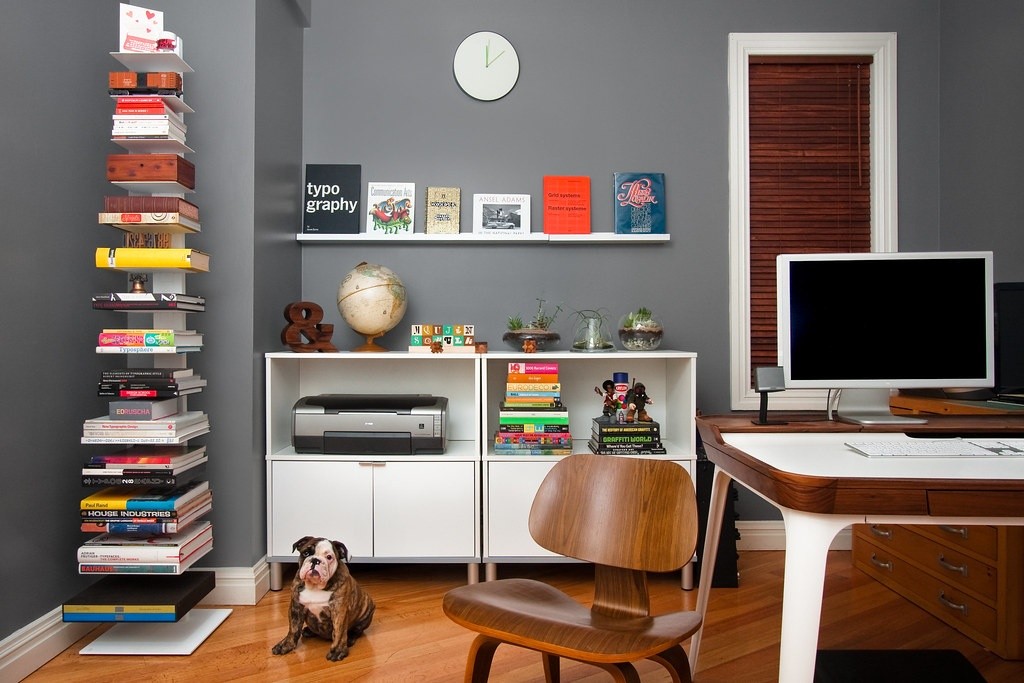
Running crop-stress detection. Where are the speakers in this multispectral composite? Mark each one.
[751,366,787,425]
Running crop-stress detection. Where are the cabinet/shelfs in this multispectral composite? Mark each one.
[851,524,1024,661]
[264,347,696,593]
[60,51,231,656]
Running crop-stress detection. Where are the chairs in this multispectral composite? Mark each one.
[442,457,703,683]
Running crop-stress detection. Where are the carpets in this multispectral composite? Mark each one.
[815,648,985,683]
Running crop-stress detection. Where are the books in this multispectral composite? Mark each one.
[97,369,208,396]
[98,196,202,233]
[303,161,361,233]
[82,446,208,487]
[81,401,210,444]
[90,291,206,313]
[80,484,212,534]
[494,363,572,456]
[110,97,188,143]
[425,187,460,234]
[589,416,667,454]
[76,522,214,575]
[544,176,591,233]
[95,328,205,353]
[614,172,666,233]
[95,246,213,273]
[366,181,415,233]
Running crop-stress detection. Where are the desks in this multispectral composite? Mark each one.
[698,411,1023,682]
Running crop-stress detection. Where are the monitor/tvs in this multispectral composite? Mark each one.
[776,250,994,425]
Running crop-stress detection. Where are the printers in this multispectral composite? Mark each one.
[290,393,449,455]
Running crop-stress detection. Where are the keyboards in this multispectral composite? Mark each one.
[845,441,1024,459]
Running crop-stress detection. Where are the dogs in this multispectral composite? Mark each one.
[272,536,377,662]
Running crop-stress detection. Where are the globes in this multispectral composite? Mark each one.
[336,262,407,353]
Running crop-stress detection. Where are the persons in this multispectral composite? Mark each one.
[595,380,618,423]
[625,382,655,422]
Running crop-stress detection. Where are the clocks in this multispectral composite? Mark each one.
[453,31,519,102]
